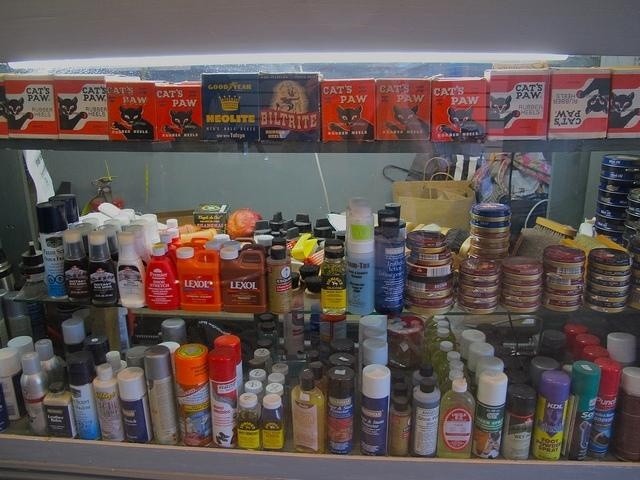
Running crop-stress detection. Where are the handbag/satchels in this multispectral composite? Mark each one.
[395,156,477,227]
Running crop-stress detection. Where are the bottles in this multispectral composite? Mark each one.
[1,301,640,461]
[34,187,177,301]
[260,199,408,314]
